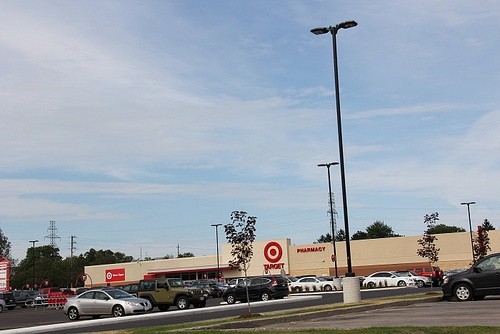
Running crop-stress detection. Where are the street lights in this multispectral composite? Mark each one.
[461,201,477,264]
[29,239,39,290]
[210,223,222,282]
[310,18,359,277]
[315,162,341,278]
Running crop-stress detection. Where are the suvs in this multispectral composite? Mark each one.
[223,275,289,304]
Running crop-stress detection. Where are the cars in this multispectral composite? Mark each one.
[440,252,500,302]
[0,265,470,312]
[63,287,152,321]
